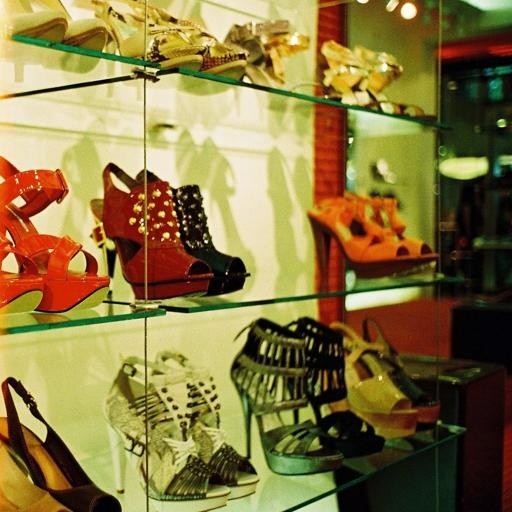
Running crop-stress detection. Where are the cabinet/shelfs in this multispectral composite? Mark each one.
[346,352,499,512]
[0,2,467,512]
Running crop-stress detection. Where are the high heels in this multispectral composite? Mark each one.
[1,0,68,41]
[329,322,419,439]
[9,0,109,83]
[273,34,342,109]
[283,317,385,457]
[361,315,441,424]
[344,189,439,259]
[179,19,248,96]
[104,356,231,512]
[309,197,419,275]
[0,155,110,314]
[224,20,295,94]
[0,377,123,512]
[0,229,44,313]
[356,42,437,124]
[93,0,208,72]
[151,350,260,500]
[230,318,344,475]
[322,41,411,120]
[1,439,74,512]
[90,163,213,299]
[136,169,251,297]
